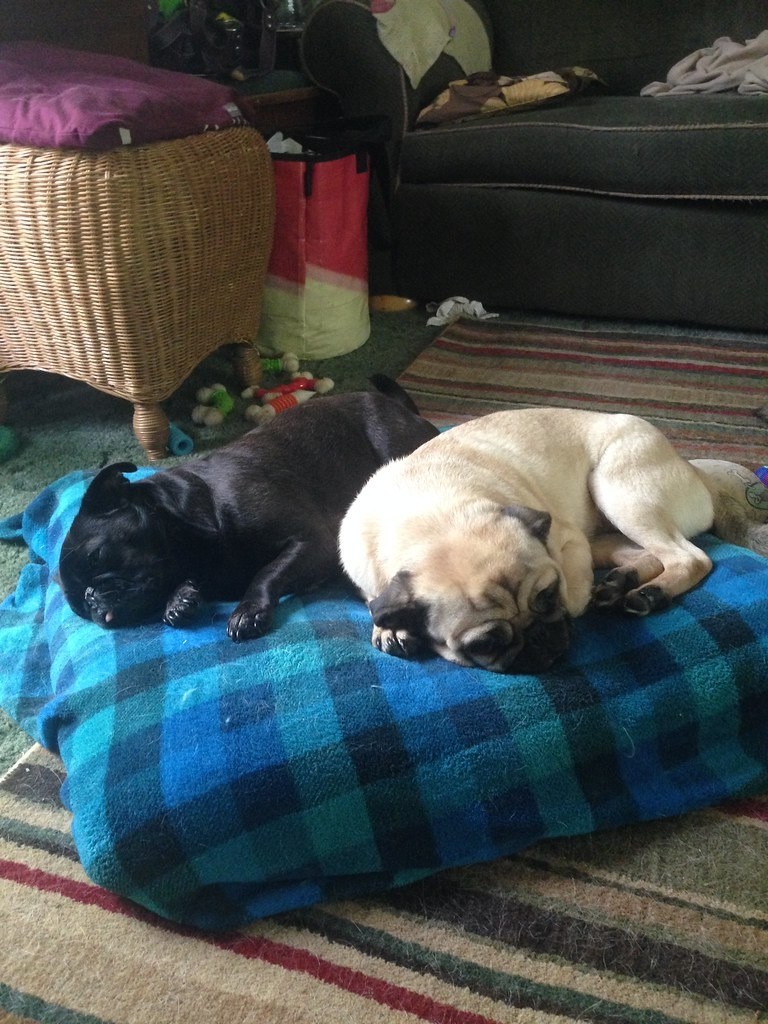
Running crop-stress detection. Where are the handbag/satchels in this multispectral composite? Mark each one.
[255,130,371,361]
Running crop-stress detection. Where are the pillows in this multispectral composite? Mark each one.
[411,62,612,129]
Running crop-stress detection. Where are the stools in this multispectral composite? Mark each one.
[0,127,286,466]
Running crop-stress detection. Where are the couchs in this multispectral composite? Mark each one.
[301,0,768,340]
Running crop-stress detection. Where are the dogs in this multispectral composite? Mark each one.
[59,388,768,675]
